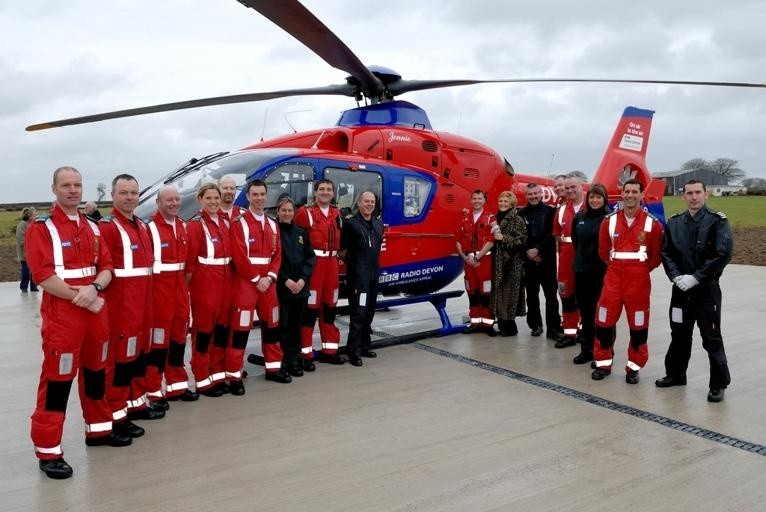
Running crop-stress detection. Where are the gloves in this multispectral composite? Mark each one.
[675,273,698,290]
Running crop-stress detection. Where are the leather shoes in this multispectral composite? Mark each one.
[500,331,512,337]
[554,335,575,348]
[265,372,291,384]
[482,325,497,337]
[303,360,315,371]
[218,382,230,391]
[655,376,688,387]
[495,330,504,334]
[573,351,593,363]
[546,327,562,339]
[128,407,164,419]
[591,368,612,378]
[461,325,476,333]
[153,399,169,410]
[85,433,133,447]
[168,391,199,401]
[363,350,377,358]
[38,458,73,479]
[203,388,222,397]
[113,420,145,437]
[290,365,303,377]
[319,353,345,365]
[30,288,40,292]
[707,385,726,402]
[625,370,639,383]
[21,288,28,293]
[230,380,245,394]
[531,325,543,336]
[351,355,363,367]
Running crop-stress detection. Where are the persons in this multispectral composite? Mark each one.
[655,179,734,403]
[83,201,102,221]
[274,194,316,376]
[26,166,133,479]
[455,189,500,337]
[592,179,665,384]
[493,191,529,337]
[100,173,165,438]
[15,206,40,293]
[185,182,232,399]
[571,183,612,364]
[518,181,565,340]
[217,174,248,387]
[292,179,346,371]
[145,186,199,410]
[341,189,384,367]
[548,173,567,211]
[225,179,292,396]
[553,177,586,349]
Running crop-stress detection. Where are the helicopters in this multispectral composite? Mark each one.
[25,0,761,364]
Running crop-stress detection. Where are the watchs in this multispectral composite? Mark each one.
[267,276,272,284]
[474,257,480,263]
[92,282,102,293]
